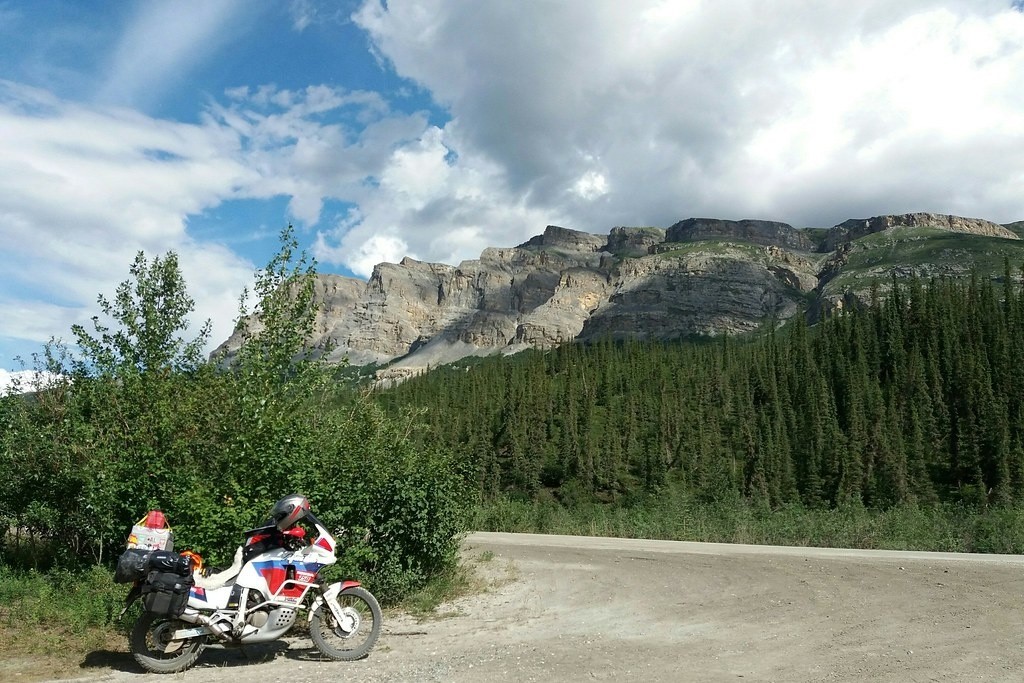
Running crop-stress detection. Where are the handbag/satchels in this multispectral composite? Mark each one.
[113,548,190,584]
[142,571,195,617]
[127,511,174,551]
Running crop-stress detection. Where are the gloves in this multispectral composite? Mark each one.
[199,568,212,577]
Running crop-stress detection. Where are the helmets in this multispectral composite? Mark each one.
[272,495,310,532]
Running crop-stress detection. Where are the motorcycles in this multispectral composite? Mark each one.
[114,494,382,674]
